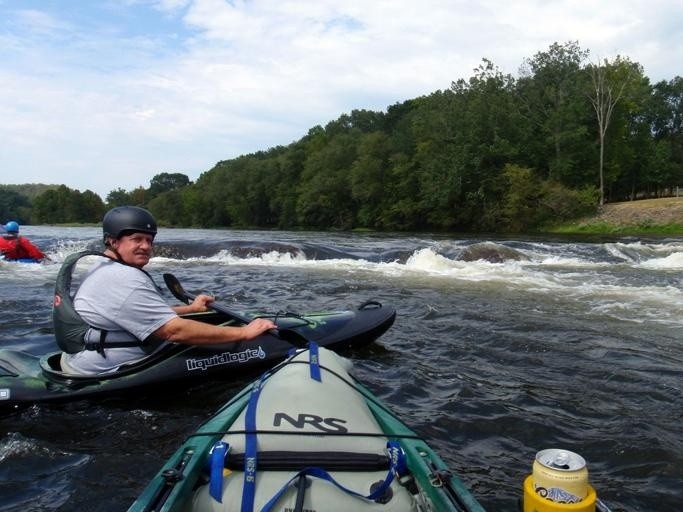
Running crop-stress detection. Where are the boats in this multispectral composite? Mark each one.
[0,248,56,265]
[0,300,397,407]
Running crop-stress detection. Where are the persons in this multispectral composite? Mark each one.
[60,206,278,377]
[0,221,44,259]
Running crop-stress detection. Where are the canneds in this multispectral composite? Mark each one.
[531,448,589,505]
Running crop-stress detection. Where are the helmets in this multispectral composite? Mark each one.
[6,222,19,233]
[103,206,157,237]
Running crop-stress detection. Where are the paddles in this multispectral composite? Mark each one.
[163,271,307,347]
